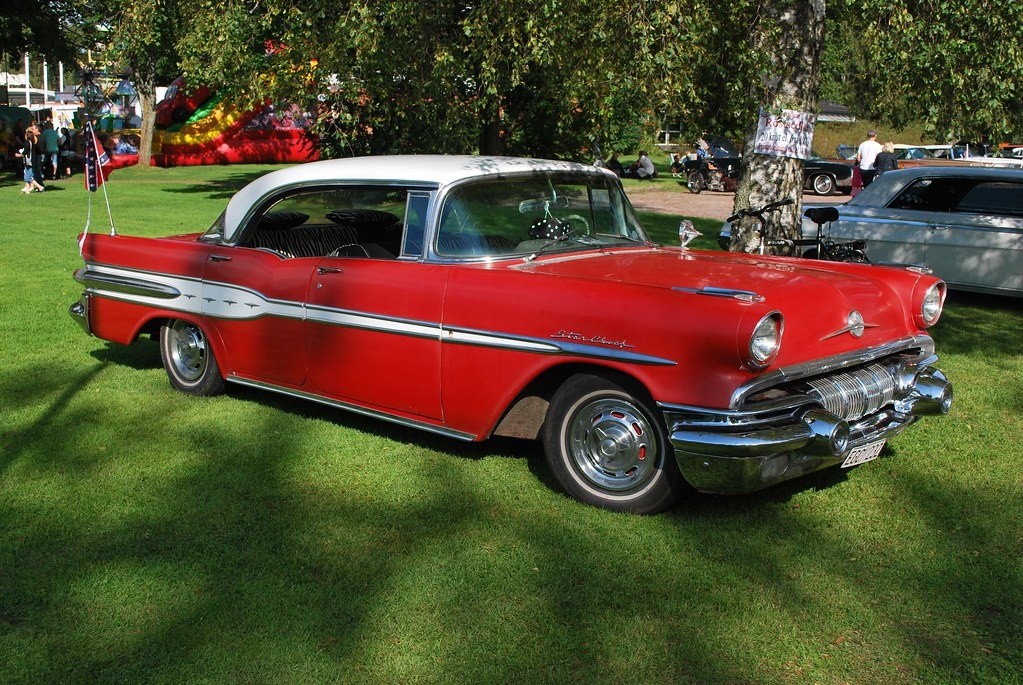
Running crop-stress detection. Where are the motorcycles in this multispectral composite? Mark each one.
[687,148,741,194]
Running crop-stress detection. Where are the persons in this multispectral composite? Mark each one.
[850,158,863,199]
[857,130,883,189]
[592,149,656,180]
[0,116,72,194]
[873,141,898,182]
[671,150,694,177]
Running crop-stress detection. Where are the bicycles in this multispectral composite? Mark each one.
[726,196,875,266]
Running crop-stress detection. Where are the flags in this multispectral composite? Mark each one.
[84,121,117,192]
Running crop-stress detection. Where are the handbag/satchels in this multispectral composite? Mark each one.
[26,157,32,167]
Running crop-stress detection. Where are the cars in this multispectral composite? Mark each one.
[716,164,1023,300]
[67,152,955,518]
[836,136,1023,187]
[684,142,856,196]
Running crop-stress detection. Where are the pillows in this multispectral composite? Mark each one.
[326,209,401,227]
[262,210,310,231]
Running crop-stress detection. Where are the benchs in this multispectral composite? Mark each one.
[249,225,513,259]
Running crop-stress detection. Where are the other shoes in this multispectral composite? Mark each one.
[29,187,34,192]
[21,187,29,192]
[36,187,46,192]
